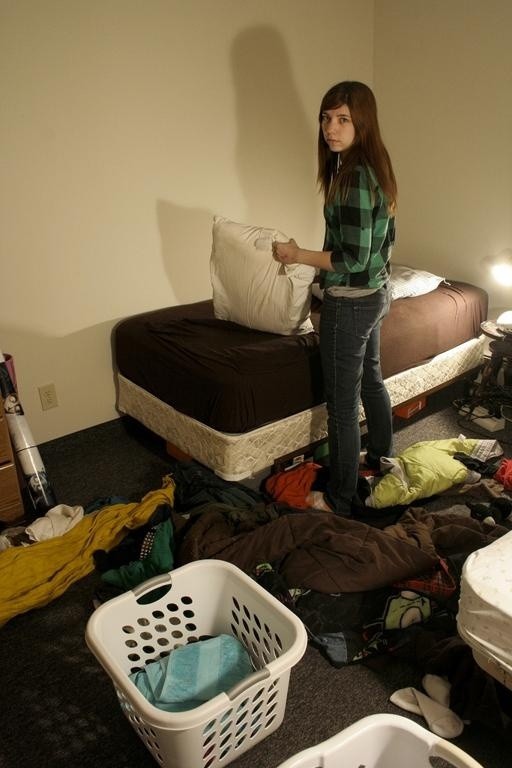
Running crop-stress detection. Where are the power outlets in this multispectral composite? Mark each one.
[37,382,60,413]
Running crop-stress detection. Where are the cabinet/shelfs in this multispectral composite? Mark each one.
[0,396,29,531]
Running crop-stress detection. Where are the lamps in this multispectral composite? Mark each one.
[489,258,512,335]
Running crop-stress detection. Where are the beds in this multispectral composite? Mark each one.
[111,277,490,484]
[454,528,511,696]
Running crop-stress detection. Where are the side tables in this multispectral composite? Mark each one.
[479,318,512,399]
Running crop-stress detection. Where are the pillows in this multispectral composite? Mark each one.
[312,261,453,304]
[208,213,319,338]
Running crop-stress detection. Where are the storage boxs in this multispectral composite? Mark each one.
[394,396,427,420]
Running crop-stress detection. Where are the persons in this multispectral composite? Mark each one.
[271,81,399,506]
